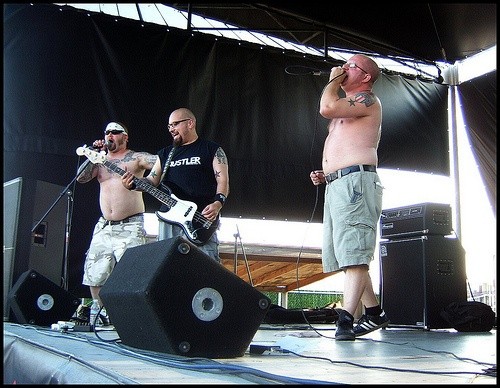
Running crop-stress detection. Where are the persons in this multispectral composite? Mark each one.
[121,108,229,263]
[76,122,157,325]
[310,54,391,342]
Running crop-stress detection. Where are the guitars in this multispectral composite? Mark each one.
[76,145,221,245]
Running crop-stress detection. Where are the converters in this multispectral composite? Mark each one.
[250,344,280,355]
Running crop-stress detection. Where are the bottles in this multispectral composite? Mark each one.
[90,300,100,327]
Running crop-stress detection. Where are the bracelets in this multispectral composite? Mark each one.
[214,193,226,206]
[128,183,136,191]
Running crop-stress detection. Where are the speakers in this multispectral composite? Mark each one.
[7,269,83,327]
[3,177,70,322]
[378,236,468,328]
[97,233,272,358]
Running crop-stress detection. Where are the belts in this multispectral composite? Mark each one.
[101,212,143,229]
[324,164,376,184]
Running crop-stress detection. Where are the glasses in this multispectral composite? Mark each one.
[168,119,190,129]
[346,63,368,74]
[104,130,126,135]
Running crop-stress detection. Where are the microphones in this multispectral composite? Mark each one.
[89,140,111,149]
[312,71,330,77]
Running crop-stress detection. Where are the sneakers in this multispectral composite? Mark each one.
[70,313,109,325]
[354,309,390,337]
[335,317,355,341]
